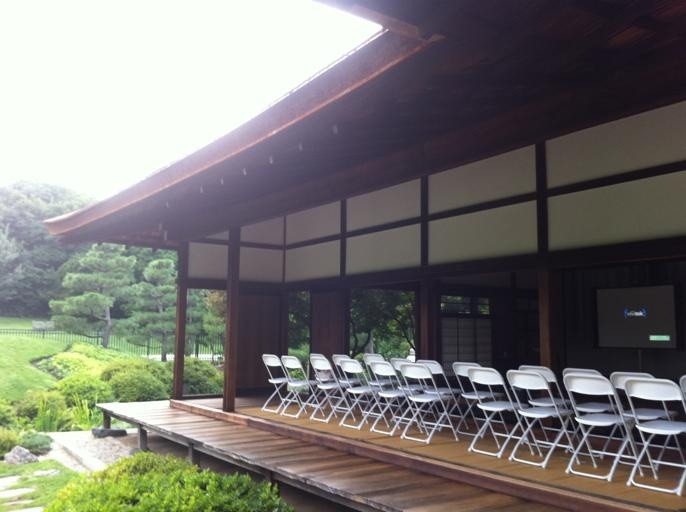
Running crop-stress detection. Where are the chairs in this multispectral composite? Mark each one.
[259,350,451,445]
[451,351,686,498]
